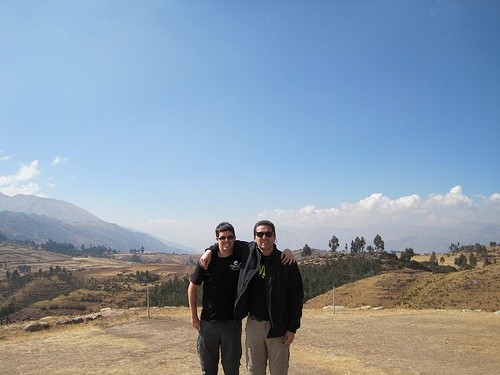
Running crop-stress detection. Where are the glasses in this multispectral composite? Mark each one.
[254,231,275,237]
[217,235,236,241]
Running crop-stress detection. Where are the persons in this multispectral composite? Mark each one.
[188,221,296,375]
[200,219,304,375]
